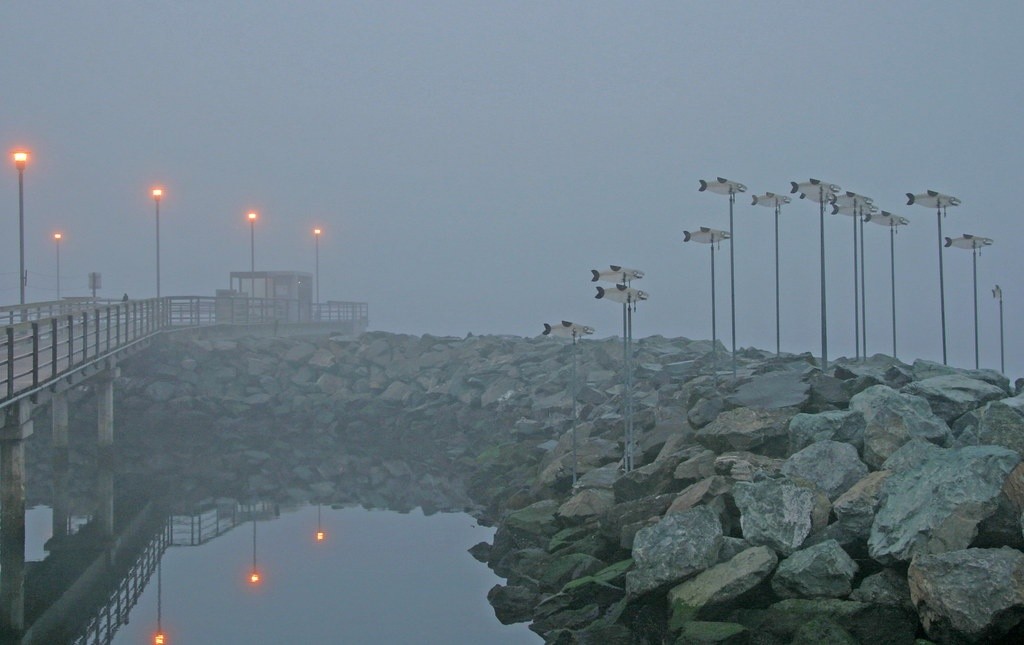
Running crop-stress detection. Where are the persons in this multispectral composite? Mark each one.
[123,294,128,301]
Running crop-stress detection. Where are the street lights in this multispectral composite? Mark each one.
[315,504,325,542]
[248,518,263,585]
[53,232,62,316]
[153,527,166,645]
[245,210,259,323]
[312,227,322,321]
[151,187,163,330]
[11,149,29,335]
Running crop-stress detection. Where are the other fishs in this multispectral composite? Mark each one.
[542,176,1003,338]
